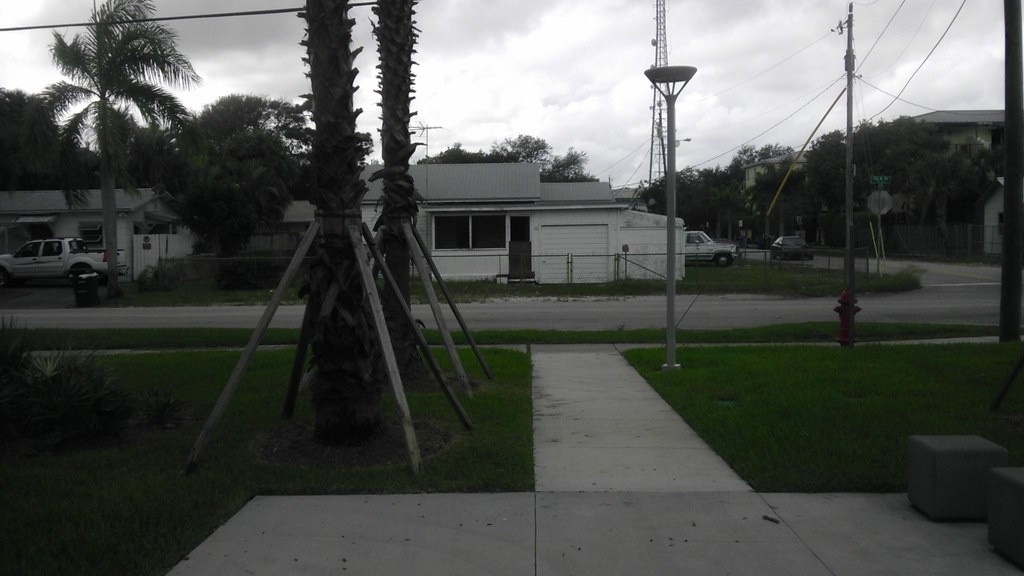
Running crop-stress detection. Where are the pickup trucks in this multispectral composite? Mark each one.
[0,237,129,288]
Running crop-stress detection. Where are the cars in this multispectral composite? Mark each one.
[771,236,815,259]
[684,231,737,264]
